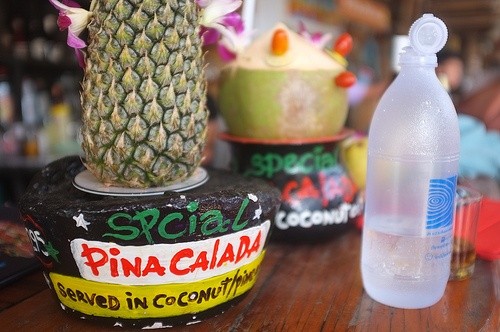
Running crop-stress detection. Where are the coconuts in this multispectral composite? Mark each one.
[218,22,356,141]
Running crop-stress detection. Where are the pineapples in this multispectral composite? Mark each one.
[79,0,210,188]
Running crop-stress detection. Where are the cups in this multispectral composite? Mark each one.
[450,183,482,279]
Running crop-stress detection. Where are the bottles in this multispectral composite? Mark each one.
[360,13,464,310]
